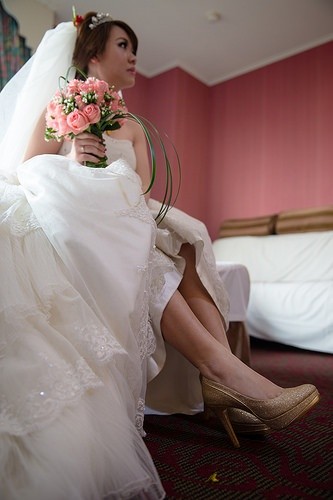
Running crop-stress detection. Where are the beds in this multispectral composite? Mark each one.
[210,228,333,354]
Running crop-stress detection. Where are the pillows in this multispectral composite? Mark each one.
[274,208,333,234]
[218,213,276,237]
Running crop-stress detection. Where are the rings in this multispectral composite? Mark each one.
[80,145,84,152]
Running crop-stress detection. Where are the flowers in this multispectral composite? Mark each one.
[73,8,83,33]
[45,66,181,226]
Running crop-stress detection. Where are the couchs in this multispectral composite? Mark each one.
[214,262,251,368]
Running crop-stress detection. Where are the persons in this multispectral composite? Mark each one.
[22,11,320,449]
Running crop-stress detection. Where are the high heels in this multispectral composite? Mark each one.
[201,373,320,448]
